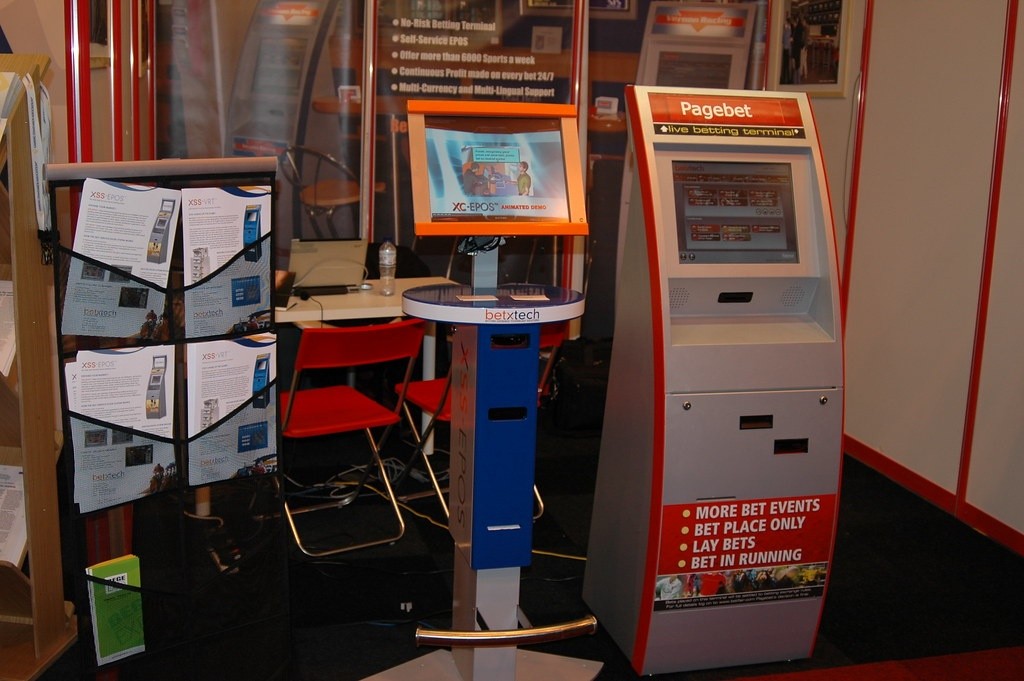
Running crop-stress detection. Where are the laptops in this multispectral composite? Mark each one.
[288,237,371,295]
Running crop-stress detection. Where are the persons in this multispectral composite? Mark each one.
[656,566,827,601]
[784,12,810,82]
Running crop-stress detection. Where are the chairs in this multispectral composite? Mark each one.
[394,324,565,521]
[280,145,388,239]
[248,319,428,557]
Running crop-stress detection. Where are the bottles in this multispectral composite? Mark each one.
[379,236,396,296]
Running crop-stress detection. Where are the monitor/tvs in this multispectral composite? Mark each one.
[405,98,588,287]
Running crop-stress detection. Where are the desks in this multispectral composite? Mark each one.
[275,276,459,456]
[312,95,448,245]
[0,54,78,681]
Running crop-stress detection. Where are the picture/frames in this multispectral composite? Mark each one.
[767,0,856,98]
[520,0,638,20]
